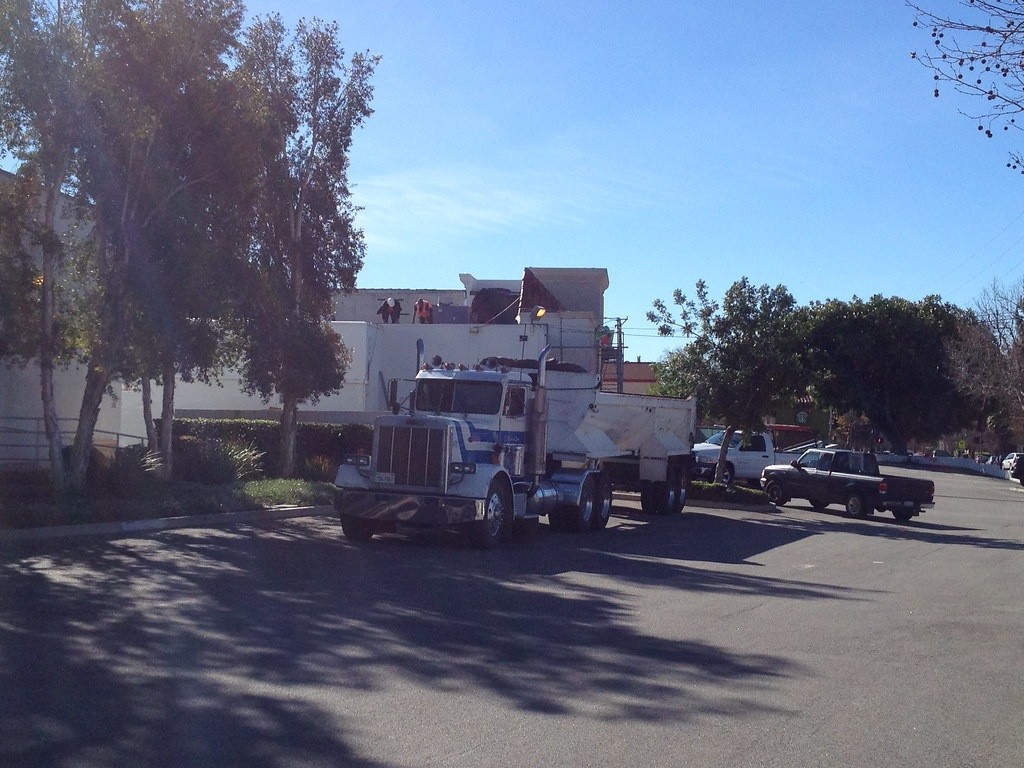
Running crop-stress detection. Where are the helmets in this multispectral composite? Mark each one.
[388,298,395,308]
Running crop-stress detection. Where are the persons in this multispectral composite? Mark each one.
[413,299,434,324]
[600,333,612,346]
[376,297,403,324]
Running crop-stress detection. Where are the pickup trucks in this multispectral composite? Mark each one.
[692,427,825,487]
[759,448,936,522]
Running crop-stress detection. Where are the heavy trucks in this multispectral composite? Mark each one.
[334,338,698,551]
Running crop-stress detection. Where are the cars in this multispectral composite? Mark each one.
[833,446,1024,482]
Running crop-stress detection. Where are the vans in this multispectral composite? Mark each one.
[762,423,817,448]
